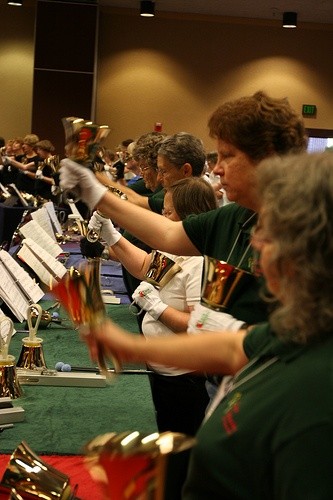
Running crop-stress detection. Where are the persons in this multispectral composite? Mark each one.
[84,151,333,500]
[0,88,310,500]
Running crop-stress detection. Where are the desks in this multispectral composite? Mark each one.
[0,224,158,500]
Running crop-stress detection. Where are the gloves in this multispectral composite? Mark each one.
[51,185,58,196]
[59,158,108,211]
[34,169,43,180]
[19,163,28,174]
[186,303,247,336]
[2,156,11,165]
[88,211,122,247]
[132,280,168,321]
